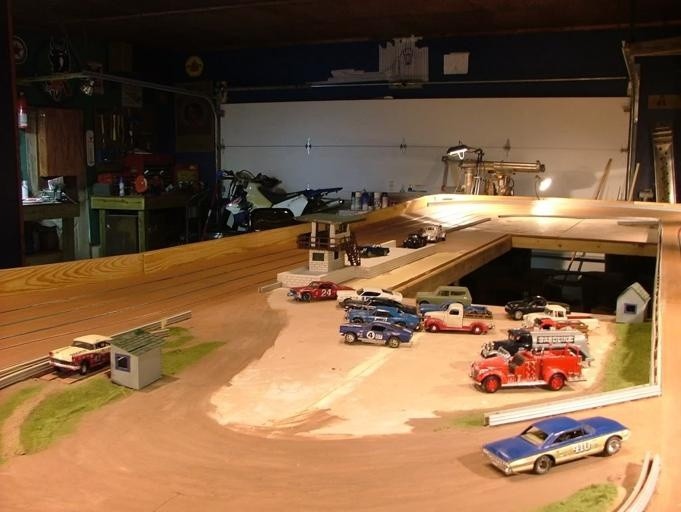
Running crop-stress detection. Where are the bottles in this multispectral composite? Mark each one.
[118,175,126,196]
[22,179,28,201]
[350,191,396,211]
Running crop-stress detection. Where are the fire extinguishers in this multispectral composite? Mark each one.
[16,91,29,129]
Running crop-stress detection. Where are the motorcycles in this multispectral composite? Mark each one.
[199,168,346,243]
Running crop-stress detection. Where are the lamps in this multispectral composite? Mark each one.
[533,175,553,200]
[447,142,482,195]
